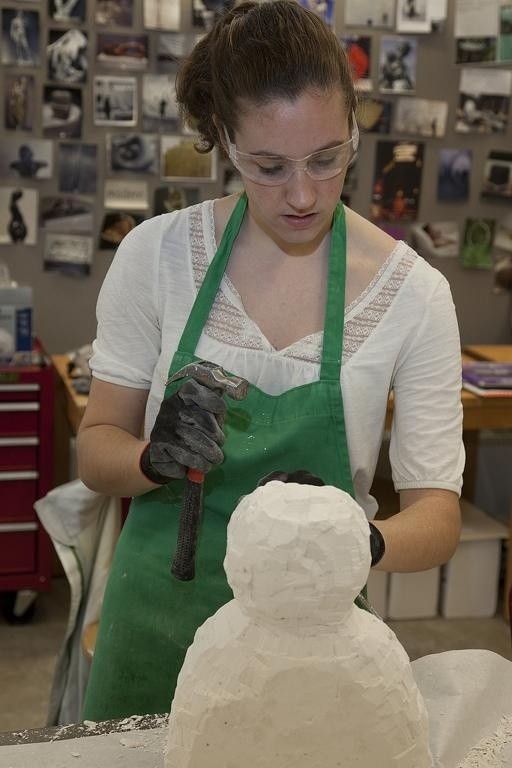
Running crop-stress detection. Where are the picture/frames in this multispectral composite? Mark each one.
[157,133,219,181]
[91,74,140,128]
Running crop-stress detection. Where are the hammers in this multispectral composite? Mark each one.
[163,361,249,581]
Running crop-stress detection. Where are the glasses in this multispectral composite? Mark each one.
[222,107,361,187]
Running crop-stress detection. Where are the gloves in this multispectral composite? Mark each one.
[139,378,228,485]
[237,470,385,568]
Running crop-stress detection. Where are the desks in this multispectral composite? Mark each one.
[382,342,511,620]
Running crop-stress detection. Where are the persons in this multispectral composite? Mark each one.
[76,0,466,723]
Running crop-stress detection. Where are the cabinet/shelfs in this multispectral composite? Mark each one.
[0,350,58,628]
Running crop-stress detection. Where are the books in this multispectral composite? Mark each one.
[462,364,512,397]
